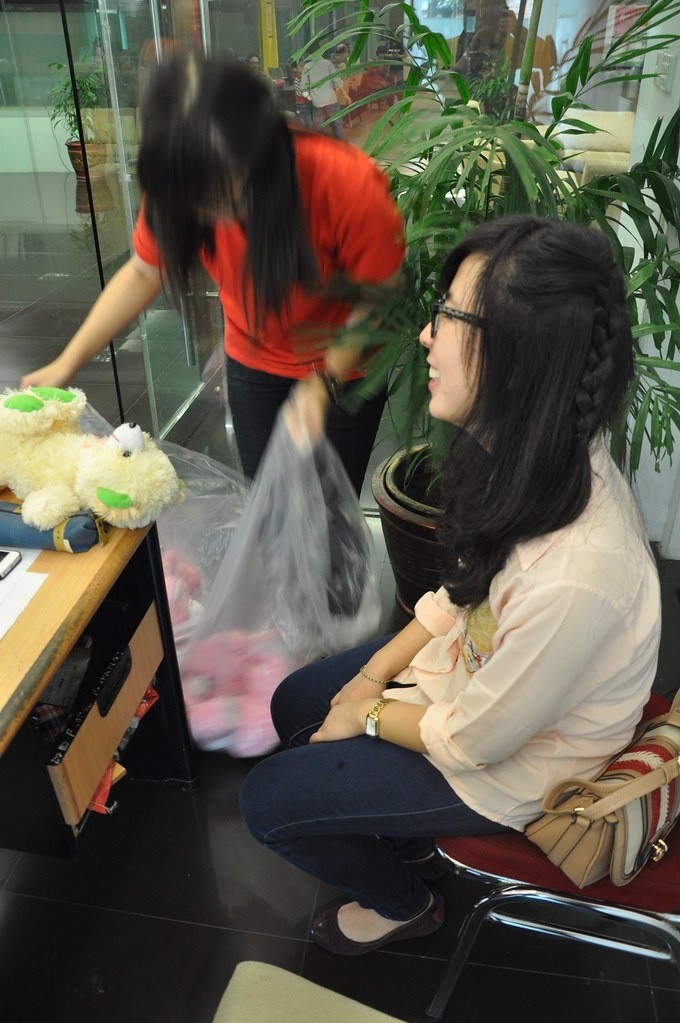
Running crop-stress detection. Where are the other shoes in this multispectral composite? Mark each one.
[399,854,447,881]
[309,889,445,955]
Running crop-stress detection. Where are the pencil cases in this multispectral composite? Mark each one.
[0,500,108,554]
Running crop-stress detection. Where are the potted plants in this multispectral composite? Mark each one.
[44,34,125,217]
[285,1,679,617]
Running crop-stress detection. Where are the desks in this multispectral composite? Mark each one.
[1,500,200,841]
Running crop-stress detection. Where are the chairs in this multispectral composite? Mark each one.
[424,691,680,1016]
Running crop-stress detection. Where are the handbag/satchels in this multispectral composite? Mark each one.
[525,691,680,890]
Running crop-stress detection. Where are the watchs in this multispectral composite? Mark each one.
[315,368,342,406]
[365,699,398,737]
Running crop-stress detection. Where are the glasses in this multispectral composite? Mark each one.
[429,293,489,340]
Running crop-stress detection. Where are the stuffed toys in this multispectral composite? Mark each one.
[161,545,304,757]
[0,386,187,531]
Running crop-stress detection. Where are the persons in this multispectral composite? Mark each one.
[291,31,408,139]
[21,54,406,616]
[241,215,663,957]
[247,54,260,71]
[437,0,556,111]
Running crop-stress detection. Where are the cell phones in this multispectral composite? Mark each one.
[0,549,21,579]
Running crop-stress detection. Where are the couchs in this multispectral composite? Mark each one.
[465,100,636,228]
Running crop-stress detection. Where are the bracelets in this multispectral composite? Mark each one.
[360,665,391,683]
